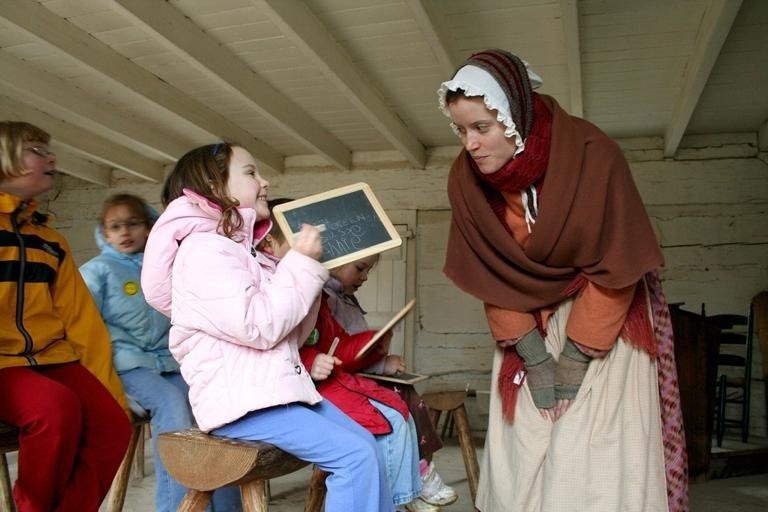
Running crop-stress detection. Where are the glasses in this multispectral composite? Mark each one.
[23,145,57,160]
[103,216,147,233]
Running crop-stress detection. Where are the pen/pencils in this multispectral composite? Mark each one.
[327,337,340,356]
[291,224,326,240]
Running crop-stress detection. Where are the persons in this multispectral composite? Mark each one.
[325,250,458,511]
[1,120,135,511]
[75,191,240,511]
[253,196,424,511]
[436,49,692,510]
[141,142,397,510]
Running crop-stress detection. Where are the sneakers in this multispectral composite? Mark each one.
[396,498,441,512]
[416,460,458,507]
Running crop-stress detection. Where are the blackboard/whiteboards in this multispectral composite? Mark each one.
[355,298,417,360]
[273,182,402,270]
[356,372,429,384]
